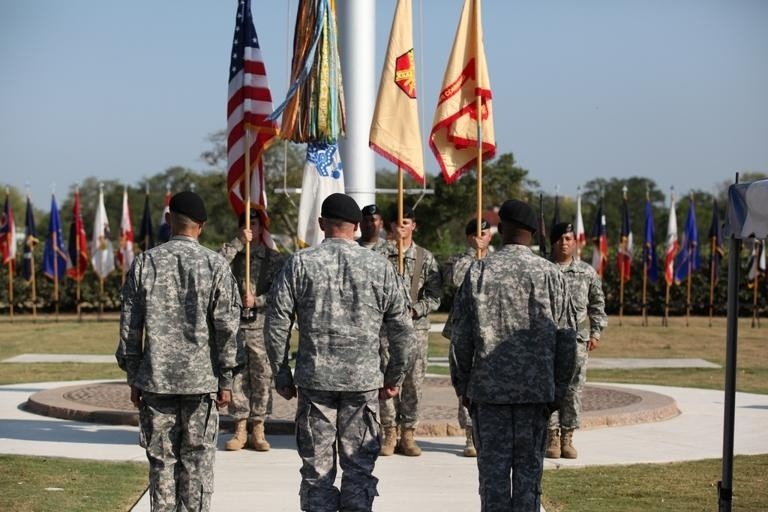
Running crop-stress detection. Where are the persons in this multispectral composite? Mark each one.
[375,204,442,456]
[544,223,608,458]
[263,193,414,512]
[443,218,492,457]
[355,204,387,250]
[218,208,286,451]
[442,199,579,512]
[115,191,243,512]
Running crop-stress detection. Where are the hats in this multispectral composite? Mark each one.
[499,200,538,230]
[466,218,491,236]
[550,223,573,246]
[169,191,209,222]
[239,208,261,227]
[322,193,362,222]
[362,205,414,218]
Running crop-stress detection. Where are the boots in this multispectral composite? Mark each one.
[252,420,271,451]
[225,419,249,451]
[545,429,577,458]
[465,429,477,457]
[400,427,421,455]
[379,427,398,455]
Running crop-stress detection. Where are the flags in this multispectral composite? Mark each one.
[226,0,282,253]
[0,188,172,282]
[297,140,362,248]
[538,195,767,288]
[429,0,496,186]
[368,0,426,186]
